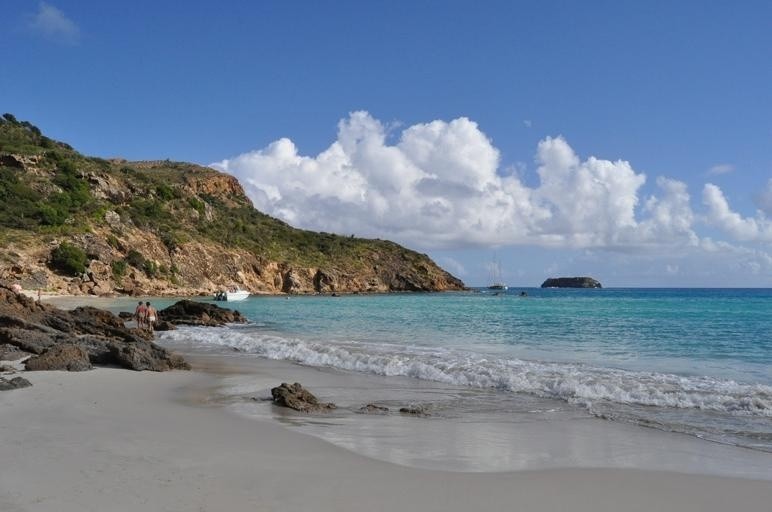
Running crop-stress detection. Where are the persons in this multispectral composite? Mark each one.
[145,301,158,330]
[134,301,146,328]
[37,289,41,300]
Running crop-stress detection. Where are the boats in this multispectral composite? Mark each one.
[213,284,251,302]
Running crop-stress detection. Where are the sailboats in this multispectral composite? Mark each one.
[485,252,509,291]
